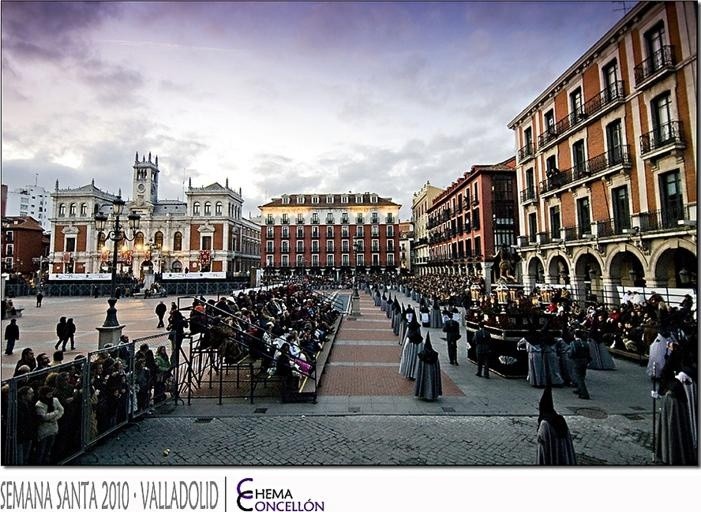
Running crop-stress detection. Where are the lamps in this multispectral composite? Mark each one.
[509,220,697,250]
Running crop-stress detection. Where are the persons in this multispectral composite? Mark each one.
[547,167,561,189]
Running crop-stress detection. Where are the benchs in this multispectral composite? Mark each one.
[176,280,345,406]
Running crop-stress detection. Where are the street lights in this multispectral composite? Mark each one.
[144,243,158,274]
[94,187,143,327]
